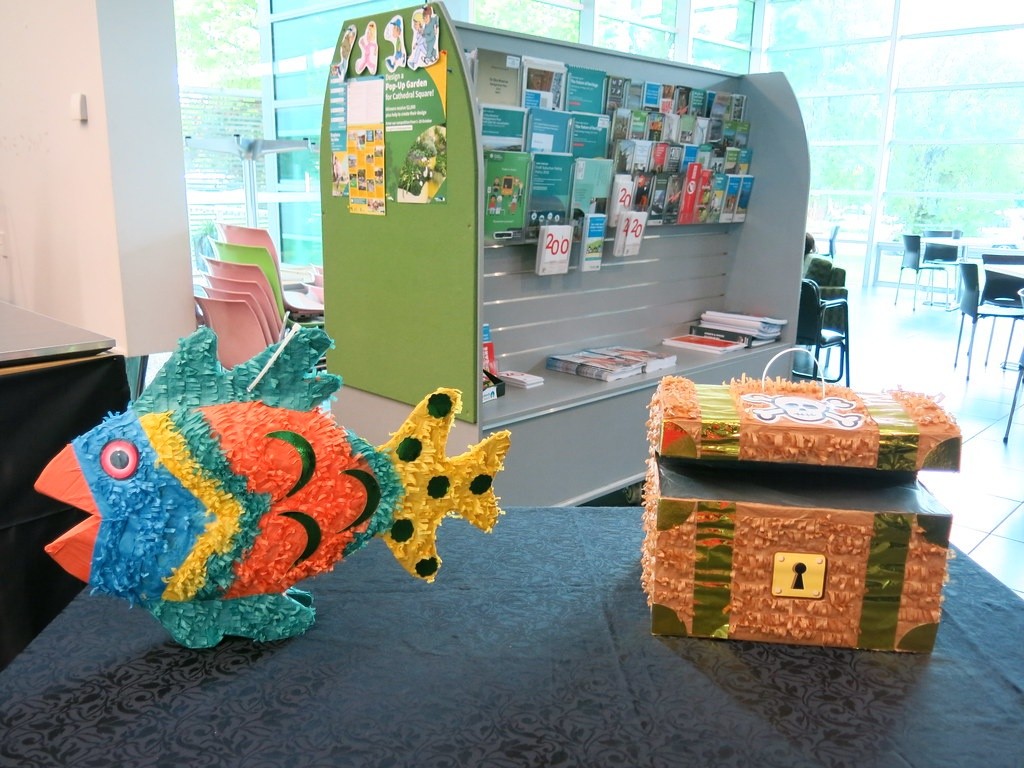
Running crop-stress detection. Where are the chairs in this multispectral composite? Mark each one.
[194,221,325,373]
[895,229,1024,381]
[793,227,849,386]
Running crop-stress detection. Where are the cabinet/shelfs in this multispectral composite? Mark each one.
[0,351,133,671]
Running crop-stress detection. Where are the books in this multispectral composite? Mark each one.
[464,44,754,246]
[482,323,545,404]
[545,345,677,382]
[612,208,649,258]
[535,225,574,276]
[578,212,607,272]
[662,308,788,354]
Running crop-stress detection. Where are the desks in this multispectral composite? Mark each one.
[985,263,1024,441]
[2,503,1024,768]
[924,237,991,303]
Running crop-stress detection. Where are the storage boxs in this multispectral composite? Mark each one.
[644,376,962,654]
